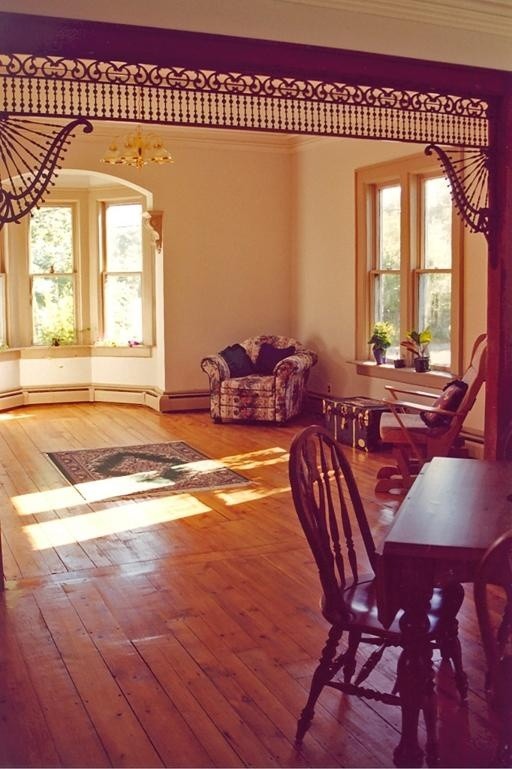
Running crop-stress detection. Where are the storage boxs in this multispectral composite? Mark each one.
[326,394,405,453]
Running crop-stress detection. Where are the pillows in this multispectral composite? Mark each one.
[420,379,468,428]
[255,343,295,376]
[218,343,256,378]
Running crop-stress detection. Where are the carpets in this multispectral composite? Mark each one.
[46,439,254,505]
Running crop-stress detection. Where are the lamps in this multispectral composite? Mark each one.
[98,125,175,170]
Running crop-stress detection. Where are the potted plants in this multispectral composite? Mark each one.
[393,352,406,368]
[399,326,432,372]
[367,320,394,364]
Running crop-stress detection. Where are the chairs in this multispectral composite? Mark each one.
[200,335,318,427]
[288,423,469,769]
[376,333,487,492]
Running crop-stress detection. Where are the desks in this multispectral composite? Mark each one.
[373,457,512,769]
